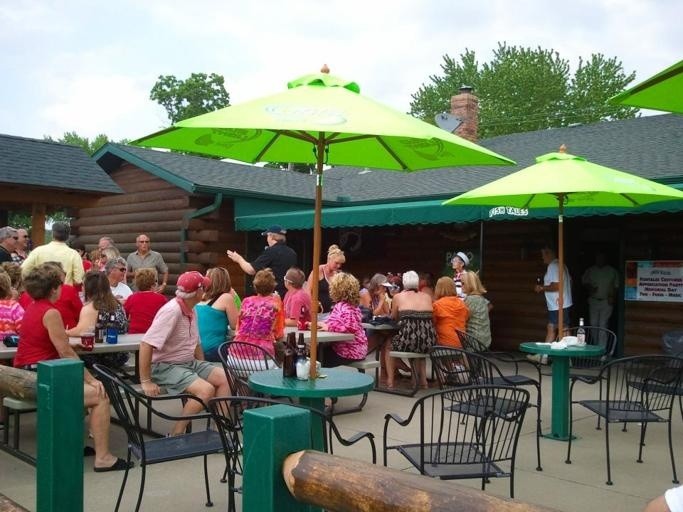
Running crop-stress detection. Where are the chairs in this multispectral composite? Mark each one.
[383,383,531,500]
[207,396,377,512]
[554,326,617,430]
[622,357,682,435]
[428,345,543,472]
[454,329,542,438]
[565,353,683,485]
[218,341,282,483]
[93,363,238,512]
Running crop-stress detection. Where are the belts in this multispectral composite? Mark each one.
[592,295,609,302]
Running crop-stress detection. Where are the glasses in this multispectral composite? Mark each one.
[388,286,400,290]
[6,236,19,241]
[19,235,29,239]
[137,240,150,245]
[284,276,294,284]
[110,266,127,273]
[100,254,105,259]
[329,250,344,256]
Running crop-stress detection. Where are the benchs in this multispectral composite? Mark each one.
[331,359,381,415]
[384,348,463,397]
[3,380,195,467]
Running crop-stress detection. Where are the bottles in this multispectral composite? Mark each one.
[296,333,306,360]
[291,332,297,349]
[106,312,117,344]
[577,318,585,345]
[298,306,305,330]
[95,312,104,342]
[283,333,296,377]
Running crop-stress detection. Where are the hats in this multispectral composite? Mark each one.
[458,251,472,265]
[260,224,288,235]
[371,273,394,289]
[176,270,211,292]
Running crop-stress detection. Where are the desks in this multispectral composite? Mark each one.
[0,332,165,360]
[519,340,606,443]
[281,324,355,342]
[359,322,395,330]
[247,367,373,453]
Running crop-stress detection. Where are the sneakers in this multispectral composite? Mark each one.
[527,354,547,365]
[215,432,244,457]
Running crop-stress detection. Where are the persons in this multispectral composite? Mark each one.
[103,258,133,304]
[227,266,285,420]
[0,226,19,264]
[306,244,346,314]
[126,232,169,295]
[71,239,93,273]
[385,270,433,389]
[451,252,470,301]
[17,261,83,331]
[317,272,368,367]
[226,224,299,301]
[124,269,168,334]
[282,267,311,330]
[581,251,621,346]
[13,262,135,474]
[138,270,235,438]
[359,273,390,383]
[433,276,470,349]
[11,228,29,262]
[459,270,494,353]
[193,266,242,362]
[387,271,403,298]
[101,246,121,270]
[20,221,85,288]
[526,243,573,366]
[66,269,129,338]
[97,236,116,254]
[0,261,25,340]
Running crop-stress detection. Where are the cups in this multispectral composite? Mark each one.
[81,333,94,352]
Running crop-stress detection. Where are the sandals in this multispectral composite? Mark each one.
[412,383,428,390]
[377,383,394,390]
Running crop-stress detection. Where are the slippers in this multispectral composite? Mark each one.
[82,446,94,457]
[92,458,135,472]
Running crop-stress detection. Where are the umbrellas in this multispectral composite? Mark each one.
[126,64,518,380]
[440,145,683,344]
[604,59,683,114]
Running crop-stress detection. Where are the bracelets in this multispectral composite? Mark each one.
[140,378,152,383]
